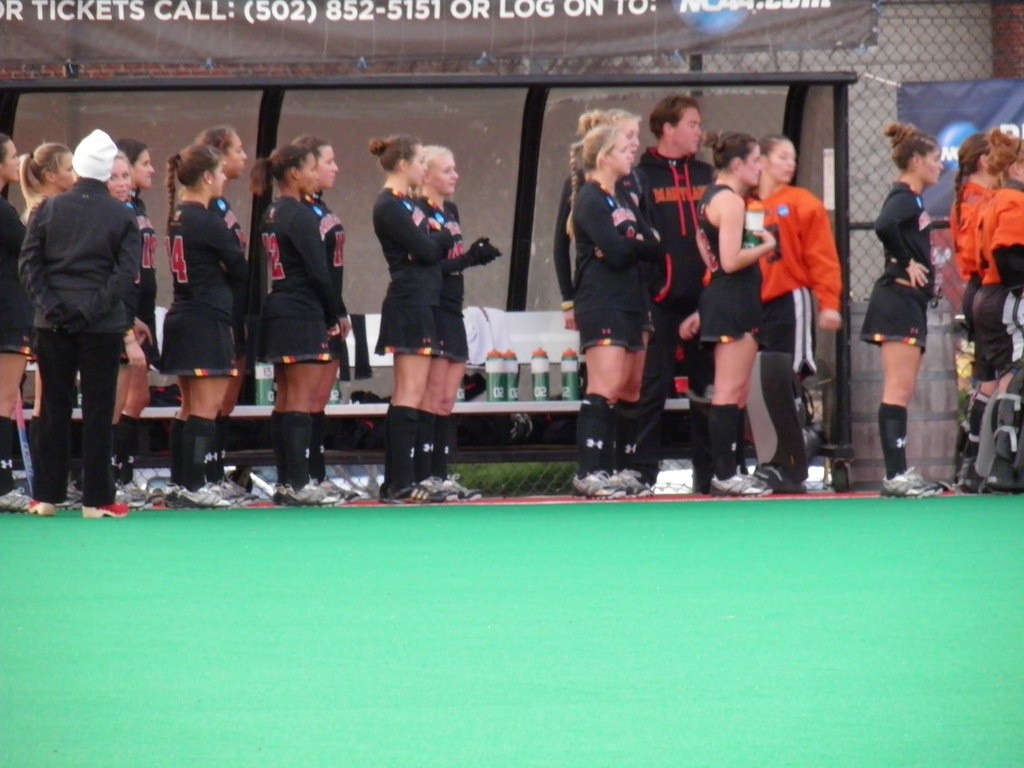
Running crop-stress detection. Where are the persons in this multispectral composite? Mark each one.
[0,131,36,511]
[17,128,165,519]
[860,122,947,496]
[164,123,362,508]
[553,93,844,499]
[368,133,503,506]
[950,128,1024,493]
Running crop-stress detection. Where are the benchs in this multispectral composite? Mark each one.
[21,305,689,427]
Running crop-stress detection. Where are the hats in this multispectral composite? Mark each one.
[71,128,118,183]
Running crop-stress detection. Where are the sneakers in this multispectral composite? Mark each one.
[376,481,448,504]
[569,468,656,499]
[0,469,361,514]
[708,464,773,498]
[880,465,944,499]
[418,472,482,501]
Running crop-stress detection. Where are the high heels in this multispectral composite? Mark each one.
[81,498,132,519]
[26,499,55,517]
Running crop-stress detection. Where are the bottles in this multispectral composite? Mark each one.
[562,300,578,331]
[560,348,580,401]
[253,360,277,405]
[326,366,341,404]
[531,348,550,401]
[742,201,764,248]
[486,347,519,402]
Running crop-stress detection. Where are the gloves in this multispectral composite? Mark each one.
[48,308,88,334]
[463,235,503,265]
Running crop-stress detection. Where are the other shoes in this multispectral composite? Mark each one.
[751,462,809,495]
[953,456,1024,494]
